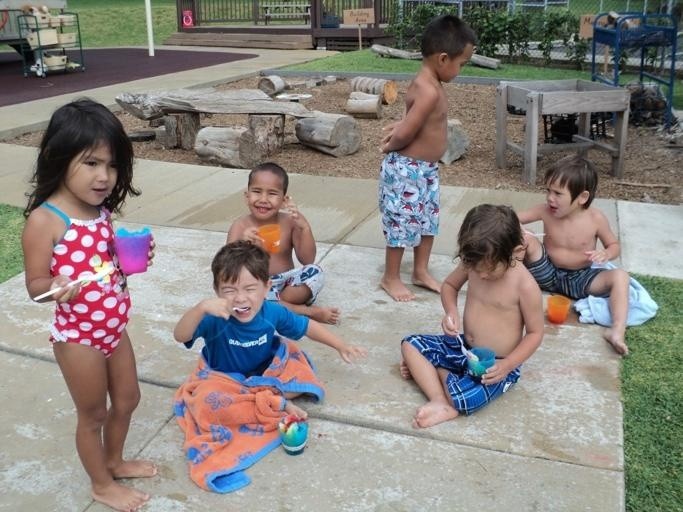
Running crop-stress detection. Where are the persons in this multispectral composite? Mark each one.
[18,96,157,512]
[174,240,368,419]
[400,203,544,430]
[225,160,342,325]
[377,14,477,302]
[514,153,629,355]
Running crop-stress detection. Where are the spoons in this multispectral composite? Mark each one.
[232,306,249,313]
[33,266,109,301]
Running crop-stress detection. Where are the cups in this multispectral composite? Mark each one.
[467,347,496,386]
[256,224,281,254]
[111,232,151,275]
[548,295,571,324]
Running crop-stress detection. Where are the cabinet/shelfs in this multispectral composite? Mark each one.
[17,12,86,79]
[592,12,678,138]
[497,79,630,184]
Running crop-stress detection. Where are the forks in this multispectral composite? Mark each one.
[448,315,479,362]
